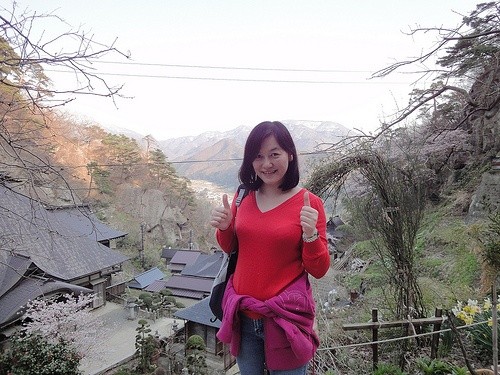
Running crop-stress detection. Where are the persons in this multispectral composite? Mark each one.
[210,120,330,375]
[360,278,366,295]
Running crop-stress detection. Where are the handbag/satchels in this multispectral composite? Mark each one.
[209,249,237,322]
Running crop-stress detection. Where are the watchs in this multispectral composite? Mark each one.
[303,227,320,243]
